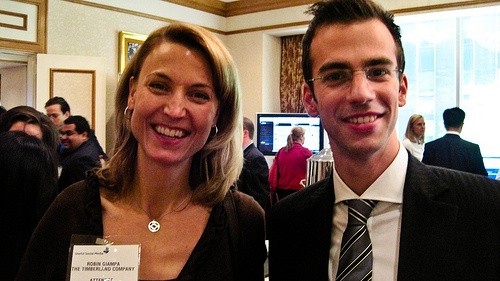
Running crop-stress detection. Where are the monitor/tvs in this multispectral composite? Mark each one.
[256,112,325,156]
[482,157,500,180]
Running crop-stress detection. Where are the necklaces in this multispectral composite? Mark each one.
[132,192,191,233]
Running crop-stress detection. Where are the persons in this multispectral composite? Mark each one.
[269,126,313,202]
[232,117,270,279]
[402,114,427,162]
[18,24,267,281]
[421,107,488,176]
[0,96,110,281]
[268,0,500,281]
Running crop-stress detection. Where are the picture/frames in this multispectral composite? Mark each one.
[50,68,96,131]
[118,31,148,75]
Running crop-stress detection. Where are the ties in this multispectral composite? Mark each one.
[336,199,379,281]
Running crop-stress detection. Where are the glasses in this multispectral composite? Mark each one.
[307,64,402,93]
